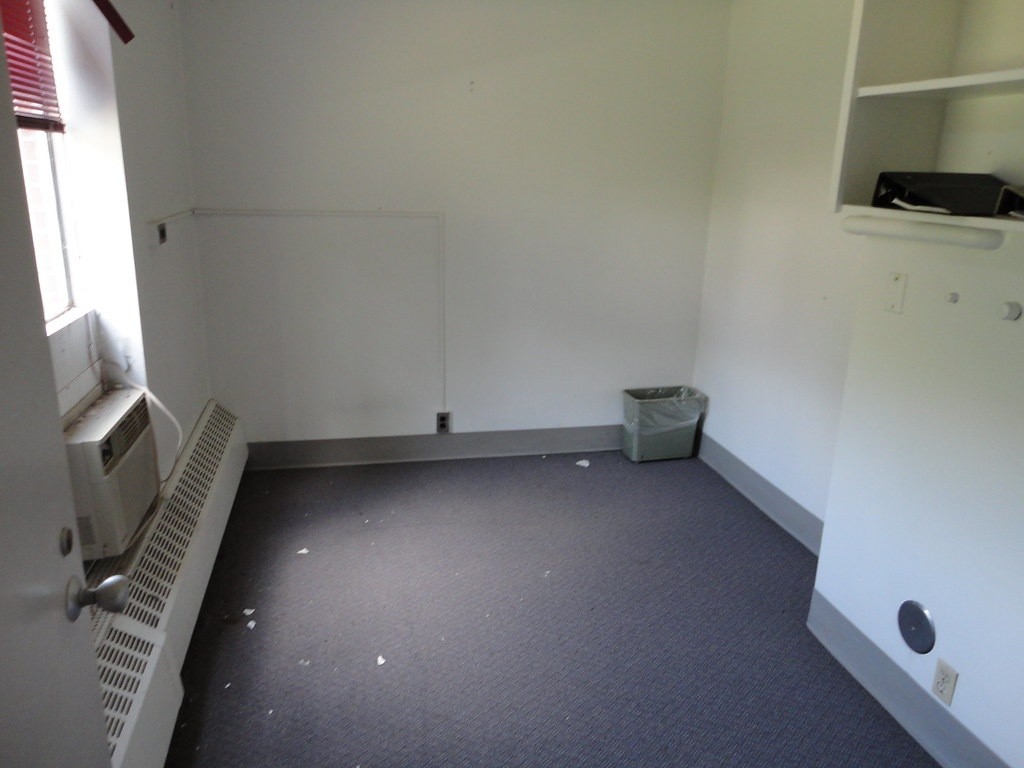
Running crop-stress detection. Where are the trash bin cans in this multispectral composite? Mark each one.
[623,386,705,463]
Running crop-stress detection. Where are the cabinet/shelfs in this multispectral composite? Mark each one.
[828,0,1024,219]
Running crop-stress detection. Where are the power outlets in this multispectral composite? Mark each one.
[932,658,958,707]
[437,413,450,432]
